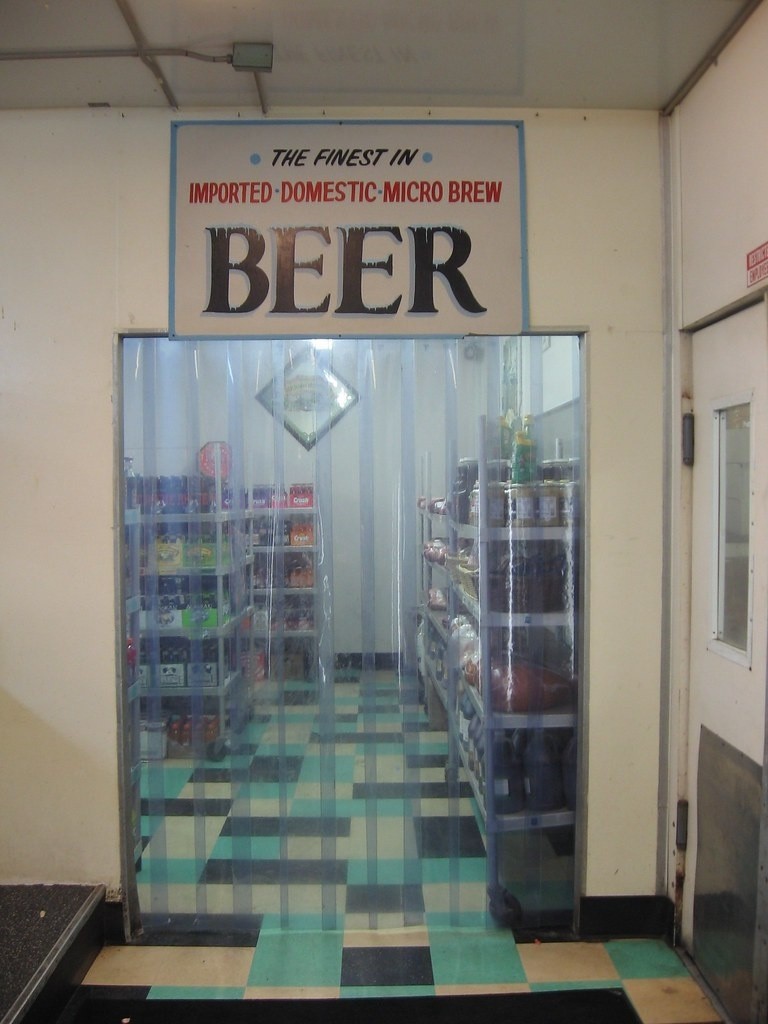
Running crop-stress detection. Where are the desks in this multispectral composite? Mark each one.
[0,883,106,1024]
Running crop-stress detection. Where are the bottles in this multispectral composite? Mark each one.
[498,414,538,484]
[430,632,578,813]
[171,717,218,746]
[124,457,313,511]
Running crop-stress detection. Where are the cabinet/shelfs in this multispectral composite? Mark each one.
[416,480,579,926]
[122,485,323,869]
